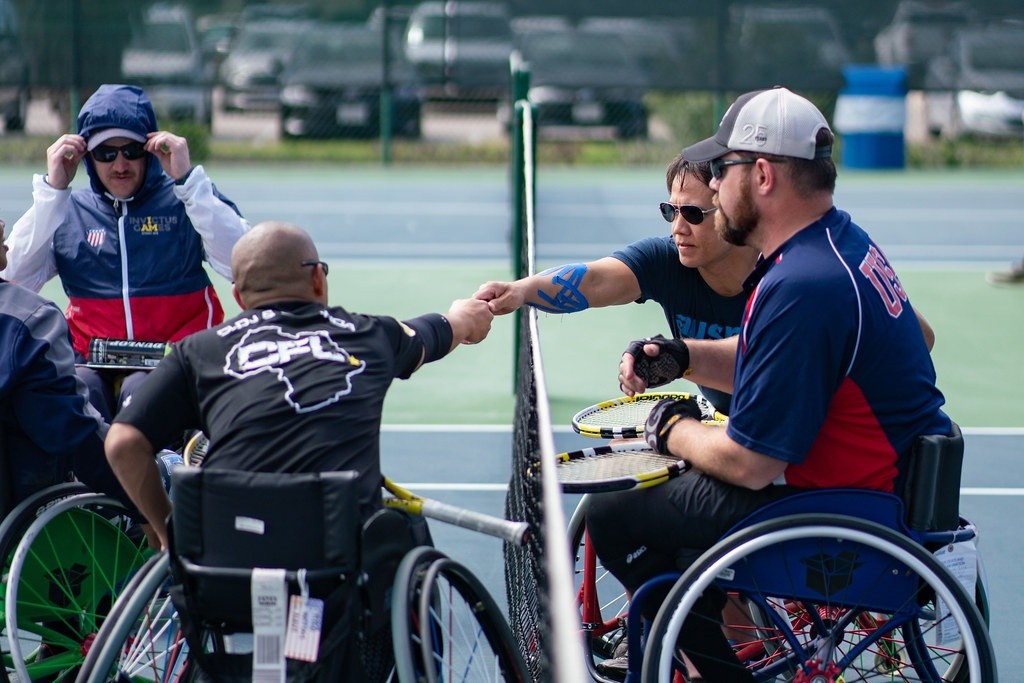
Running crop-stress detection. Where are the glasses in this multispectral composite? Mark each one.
[301,259,330,277]
[90,141,146,163]
[705,153,787,179]
[658,201,717,227]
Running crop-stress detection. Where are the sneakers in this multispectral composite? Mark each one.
[596,615,634,680]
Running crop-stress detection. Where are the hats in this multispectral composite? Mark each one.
[86,126,154,152]
[682,86,833,169]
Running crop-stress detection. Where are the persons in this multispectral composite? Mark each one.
[0,83,254,683]
[472,84,953,682]
[102,221,492,683]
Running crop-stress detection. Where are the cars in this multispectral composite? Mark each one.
[405,0,516,86]
[0,0,30,131]
[221,19,313,111]
[278,25,422,140]
[740,8,849,75]
[507,29,652,140]
[875,0,975,66]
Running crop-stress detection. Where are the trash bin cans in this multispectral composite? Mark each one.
[833,64,907,170]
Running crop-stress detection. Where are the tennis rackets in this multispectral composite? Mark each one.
[526,433,694,497]
[569,385,733,440]
[181,427,531,549]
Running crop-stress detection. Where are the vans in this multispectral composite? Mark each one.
[121,5,201,82]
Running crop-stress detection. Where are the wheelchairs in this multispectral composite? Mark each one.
[0,482,209,683]
[565,418,999,683]
[74,545,534,683]
